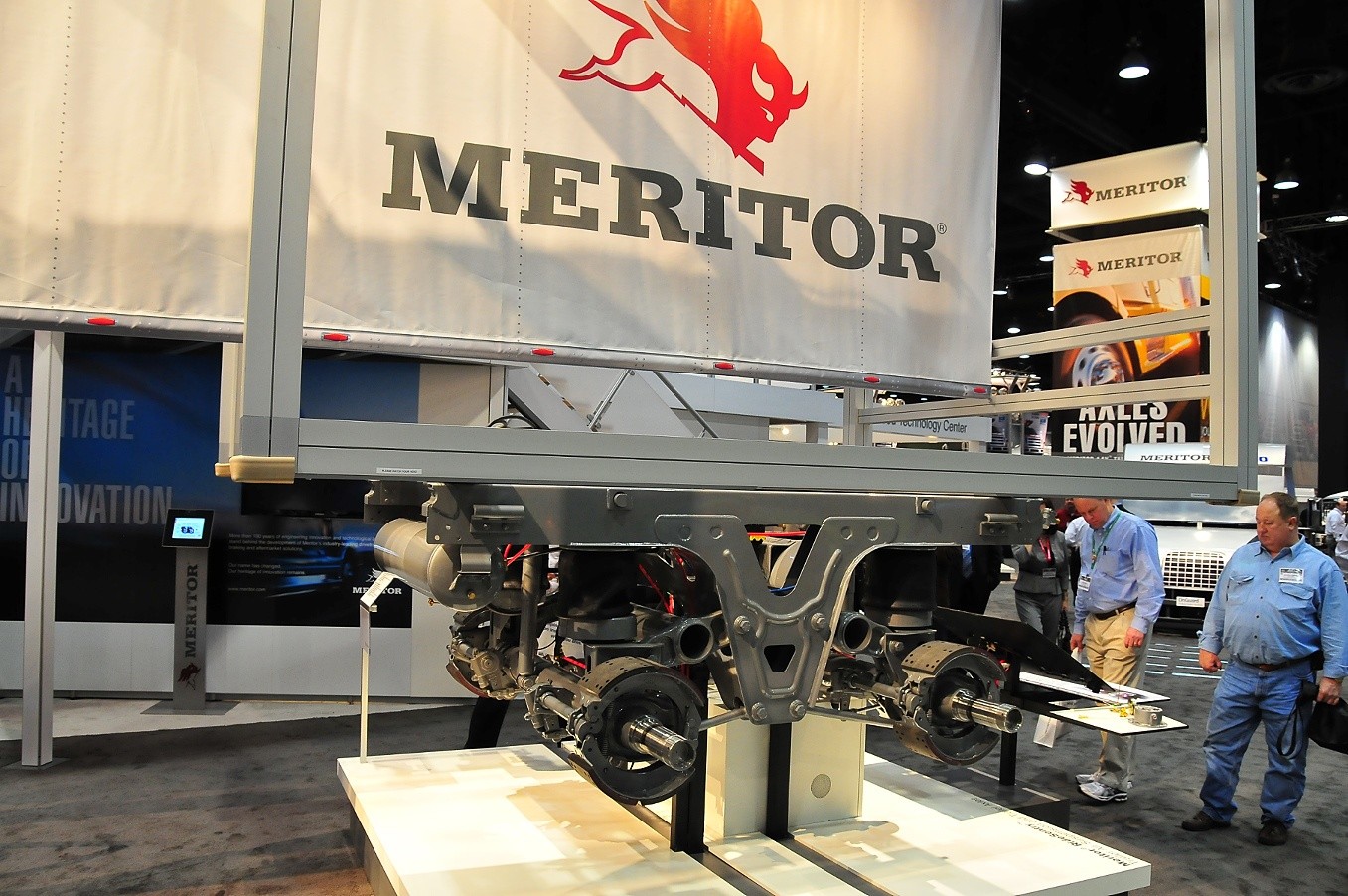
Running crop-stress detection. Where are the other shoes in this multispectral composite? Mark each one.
[1182,811,1229,832]
[1258,823,1287,847]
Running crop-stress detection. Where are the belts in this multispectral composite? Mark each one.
[1094,601,1138,620]
[1017,569,1061,579]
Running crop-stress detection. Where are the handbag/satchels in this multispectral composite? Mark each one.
[1056,610,1072,655]
[1276,679,1348,760]
[1032,647,1081,749]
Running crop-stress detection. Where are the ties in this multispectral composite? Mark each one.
[959,544,973,579]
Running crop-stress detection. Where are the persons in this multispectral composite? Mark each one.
[1010,496,1068,648]
[1069,494,1167,803]
[1325,500,1347,556]
[1335,525,1348,583]
[1181,489,1348,846]
[1055,495,1137,608]
[461,544,562,750]
[948,541,1004,615]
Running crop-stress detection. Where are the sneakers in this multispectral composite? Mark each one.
[1074,773,1098,784]
[1078,780,1127,802]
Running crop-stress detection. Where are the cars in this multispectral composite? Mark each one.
[1052,273,1193,388]
